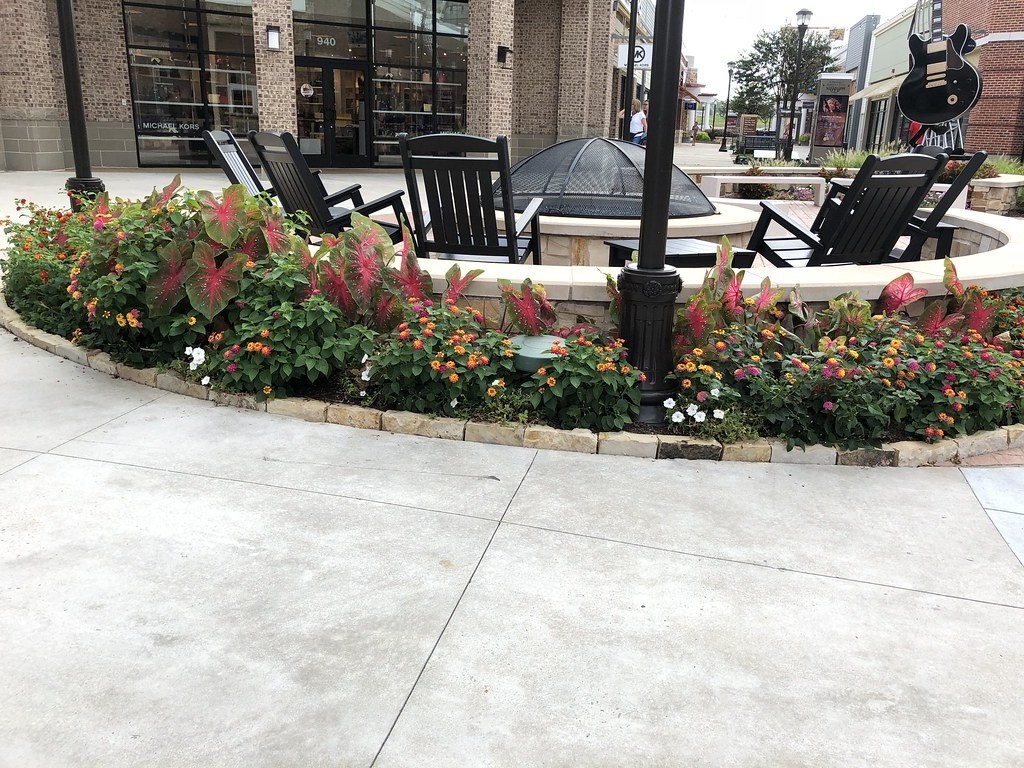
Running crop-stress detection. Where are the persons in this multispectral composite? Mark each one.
[639,99,649,145]
[690,122,699,143]
[618,98,646,145]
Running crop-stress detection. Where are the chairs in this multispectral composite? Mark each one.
[205,128,324,206]
[247,131,415,249]
[748,142,986,266]
[395,131,542,266]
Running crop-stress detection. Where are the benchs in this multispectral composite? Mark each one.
[701,176,826,206]
[829,174,969,209]
[742,136,782,156]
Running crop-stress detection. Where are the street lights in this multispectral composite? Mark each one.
[719,60,736,151]
[700,103,705,132]
[786,8,813,161]
[712,100,717,140]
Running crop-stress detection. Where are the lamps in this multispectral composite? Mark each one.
[266,25,282,52]
[498,46,513,70]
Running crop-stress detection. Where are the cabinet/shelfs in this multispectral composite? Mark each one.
[298,87,323,105]
[373,79,462,145]
[131,63,253,141]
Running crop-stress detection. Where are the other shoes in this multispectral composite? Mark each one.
[692,144,695,146]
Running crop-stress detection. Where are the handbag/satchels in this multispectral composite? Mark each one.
[630,131,644,142]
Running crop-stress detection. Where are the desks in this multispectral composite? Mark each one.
[604,239,756,270]
